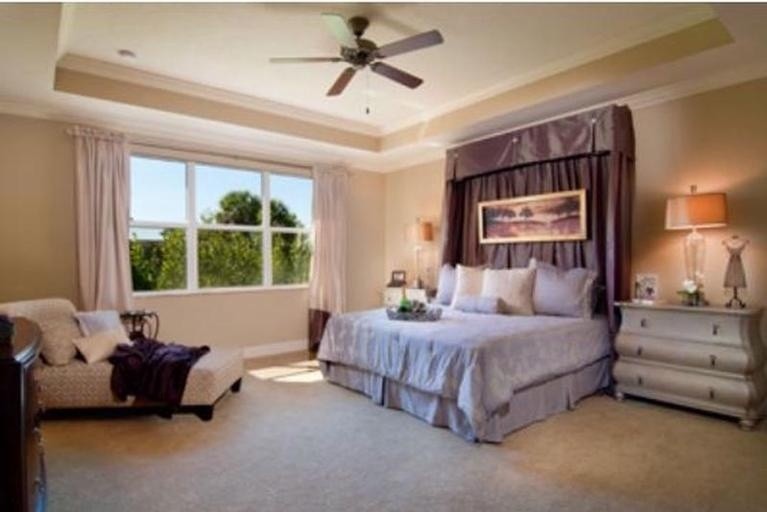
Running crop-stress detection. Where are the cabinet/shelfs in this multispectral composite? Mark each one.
[1,313,48,511]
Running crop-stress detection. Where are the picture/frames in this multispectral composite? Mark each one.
[477,187,588,245]
[391,270,407,282]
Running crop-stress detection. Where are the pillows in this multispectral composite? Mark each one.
[71,309,134,365]
[434,255,600,318]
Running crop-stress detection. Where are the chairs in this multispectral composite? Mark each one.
[0,298,244,421]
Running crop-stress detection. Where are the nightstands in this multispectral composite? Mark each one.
[611,299,767,433]
[382,287,430,309]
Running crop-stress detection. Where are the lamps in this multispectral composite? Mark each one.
[663,185,727,306]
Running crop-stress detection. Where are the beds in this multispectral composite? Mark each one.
[329,304,612,443]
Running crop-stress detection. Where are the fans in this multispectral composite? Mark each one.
[268,12,445,97]
